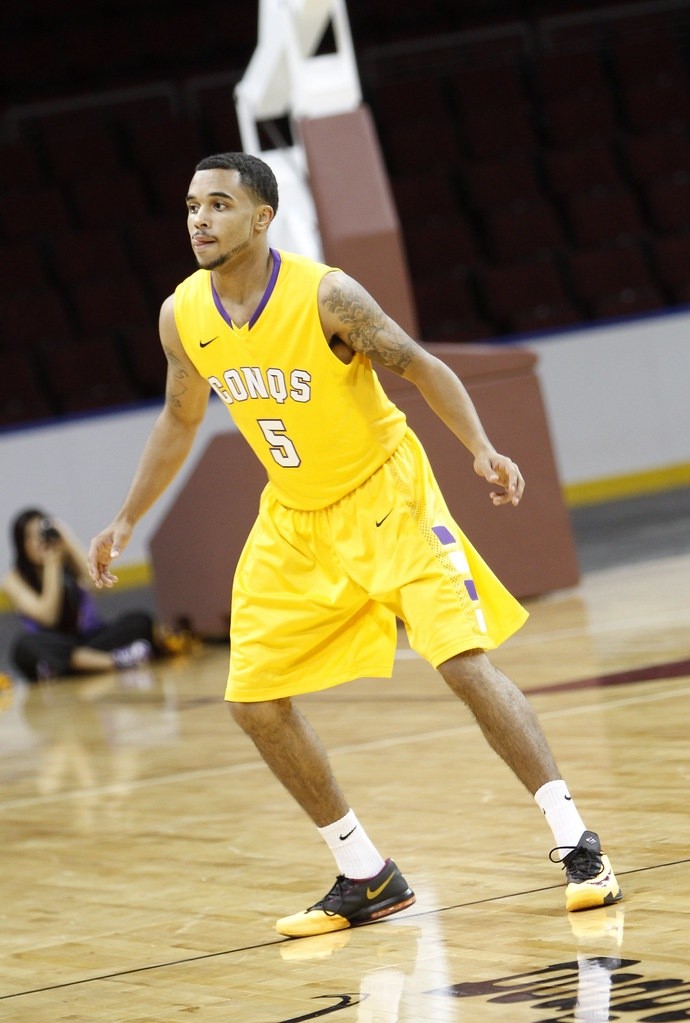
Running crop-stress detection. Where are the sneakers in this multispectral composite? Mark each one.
[548,832,619,912]
[276,855,412,938]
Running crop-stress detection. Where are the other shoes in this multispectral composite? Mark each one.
[113,641,152,672]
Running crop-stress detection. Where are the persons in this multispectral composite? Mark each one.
[87,152,622,935]
[0,506,153,685]
